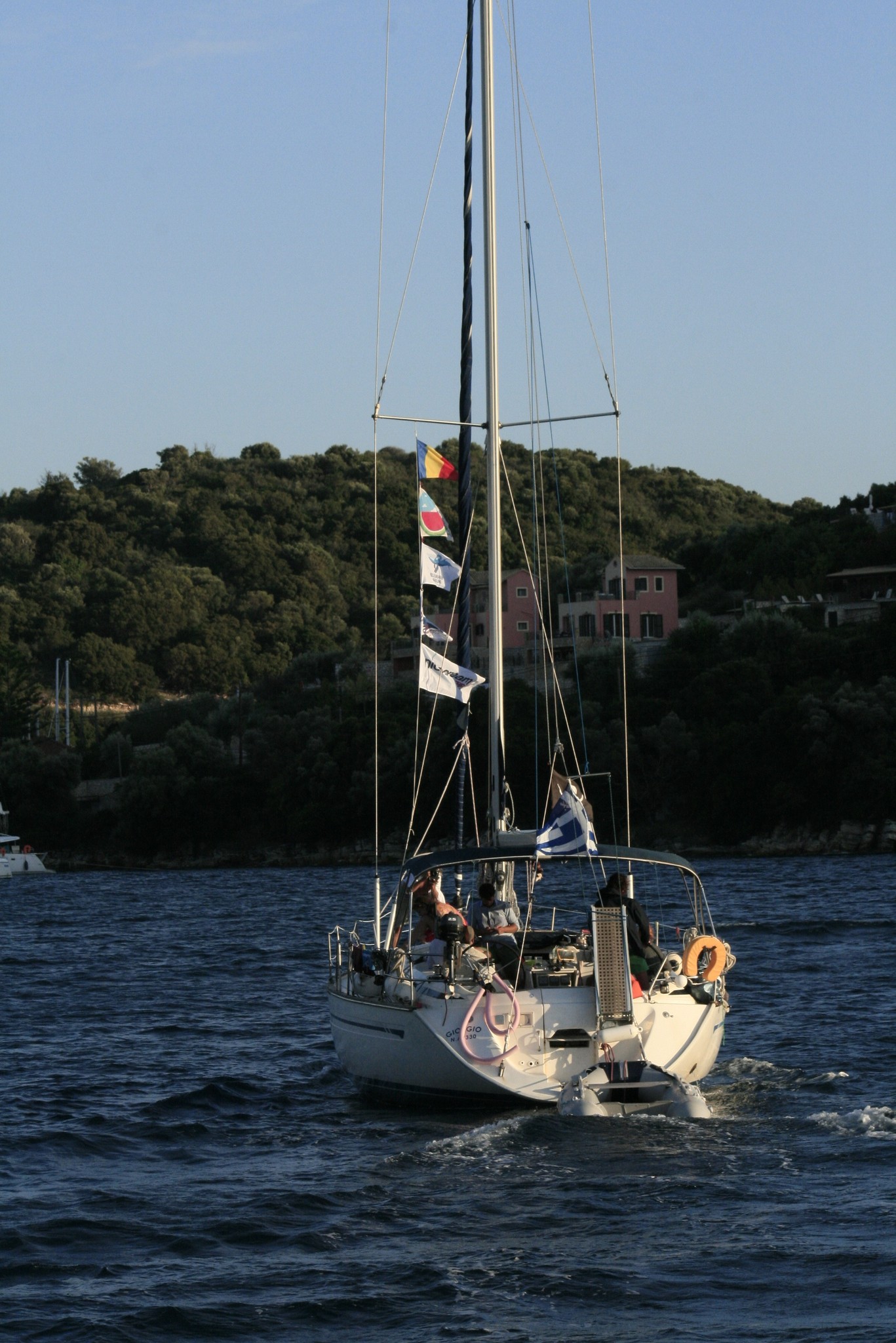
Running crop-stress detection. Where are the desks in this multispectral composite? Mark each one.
[530,960,579,987]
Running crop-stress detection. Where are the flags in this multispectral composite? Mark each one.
[419,487,454,542]
[537,783,598,855]
[421,542,461,592]
[417,438,458,482]
[421,615,453,642]
[419,642,486,703]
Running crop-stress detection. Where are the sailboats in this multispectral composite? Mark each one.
[322,0,735,1122]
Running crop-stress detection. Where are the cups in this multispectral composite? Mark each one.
[549,951,557,964]
[524,956,543,969]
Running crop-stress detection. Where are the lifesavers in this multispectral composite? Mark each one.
[24,845,31,854]
[0,848,5,855]
[682,935,726,982]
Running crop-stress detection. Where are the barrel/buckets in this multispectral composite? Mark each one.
[433,964,442,974]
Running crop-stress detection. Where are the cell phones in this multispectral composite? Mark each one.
[489,929,495,933]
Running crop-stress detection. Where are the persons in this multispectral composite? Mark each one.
[414,849,672,989]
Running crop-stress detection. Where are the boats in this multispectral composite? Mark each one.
[0,803,58,877]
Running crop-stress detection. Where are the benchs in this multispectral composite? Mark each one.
[513,931,560,957]
